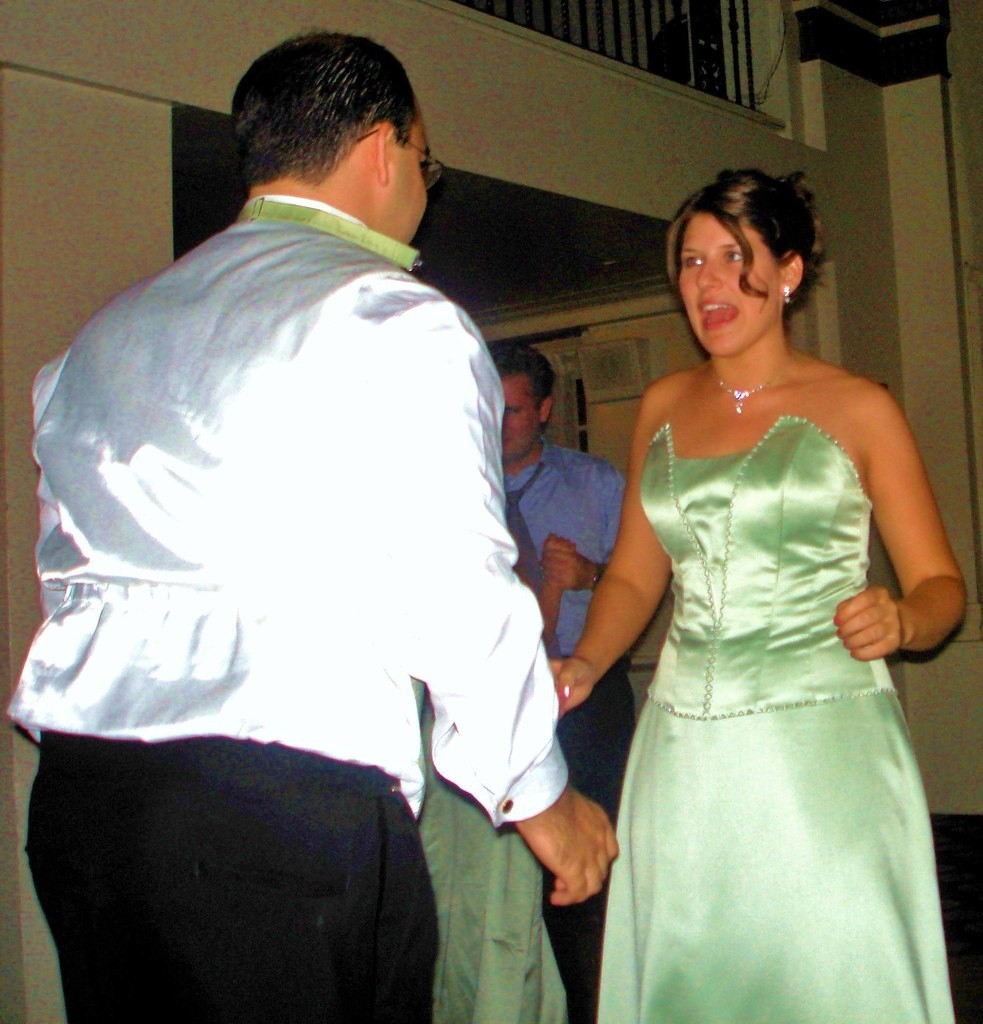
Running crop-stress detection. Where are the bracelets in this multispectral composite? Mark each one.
[594,565,604,585]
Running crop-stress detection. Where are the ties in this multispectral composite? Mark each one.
[505,462,561,660]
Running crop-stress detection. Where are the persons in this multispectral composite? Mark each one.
[488,341,633,1024]
[413,534,575,1023]
[5,29,618,1024]
[546,170,968,1024]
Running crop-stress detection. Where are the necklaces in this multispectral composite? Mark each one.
[708,366,763,414]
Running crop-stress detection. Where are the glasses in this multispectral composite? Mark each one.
[355,127,443,190]
